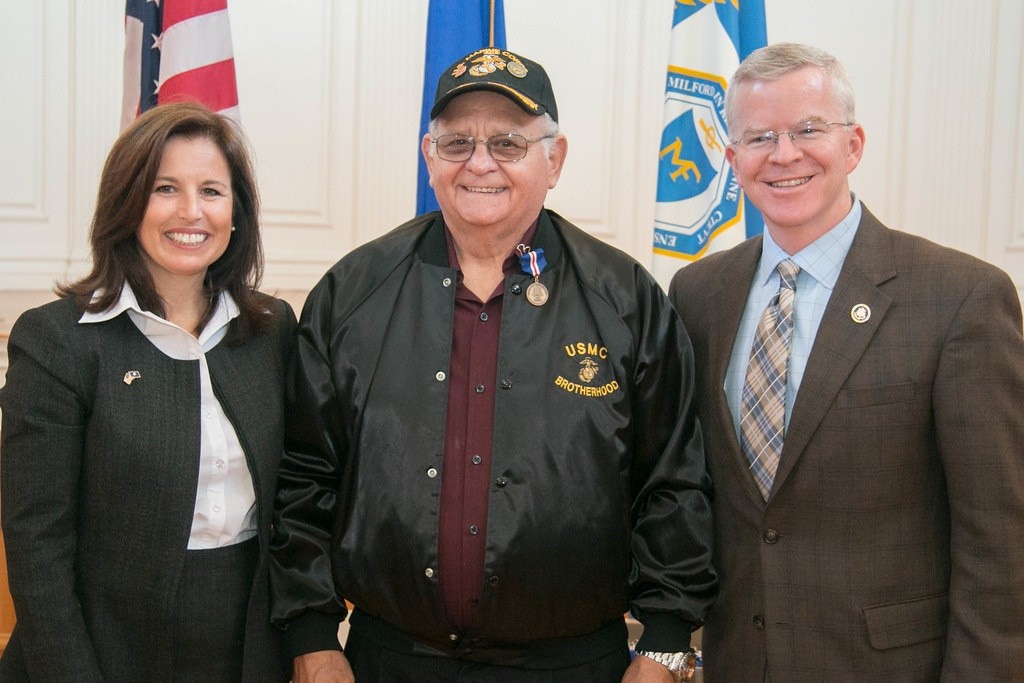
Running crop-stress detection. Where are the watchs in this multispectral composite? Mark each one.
[635,651,696,683]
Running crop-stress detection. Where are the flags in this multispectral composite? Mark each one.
[415,0,508,218]
[649,0,768,297]
[120,1,241,147]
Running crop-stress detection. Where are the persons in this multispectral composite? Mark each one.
[0,101,296,683]
[669,44,1024,683]
[271,48,718,683]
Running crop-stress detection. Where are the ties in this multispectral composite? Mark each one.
[739,259,800,503]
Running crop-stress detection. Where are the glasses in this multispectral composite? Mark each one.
[730,119,852,149]
[425,133,553,162]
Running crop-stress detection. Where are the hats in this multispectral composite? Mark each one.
[429,47,559,122]
